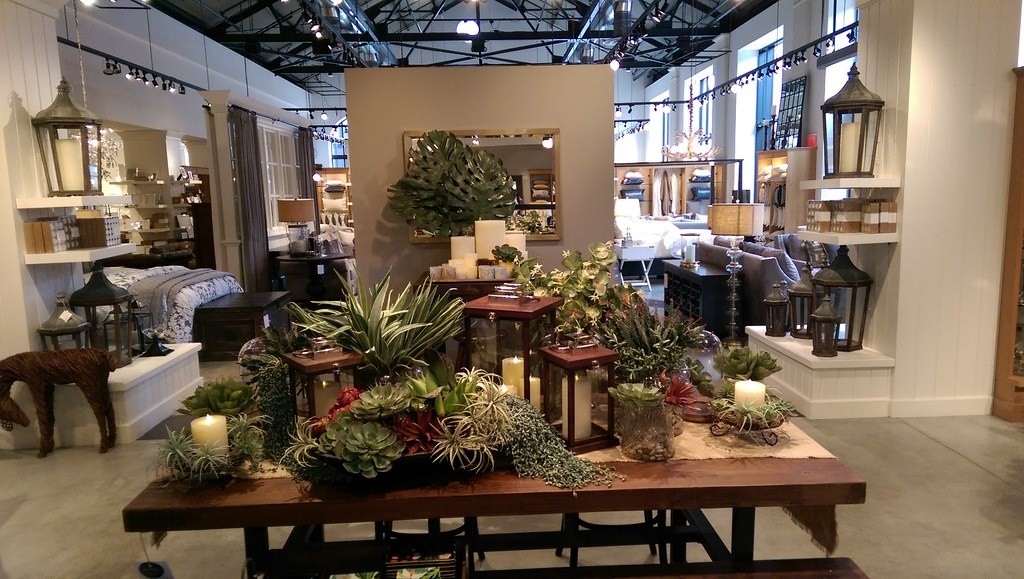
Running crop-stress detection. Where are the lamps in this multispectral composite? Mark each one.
[605,0,859,164]
[202,104,211,113]
[375,22,389,42]
[568,19,582,42]
[103,58,185,95]
[105,301,154,353]
[37,290,90,384]
[764,245,874,356]
[281,0,356,76]
[398,57,409,68]
[278,198,316,257]
[69,265,133,369]
[541,133,554,149]
[308,111,314,119]
[552,55,564,65]
[321,111,327,119]
[312,127,348,147]
[712,204,765,350]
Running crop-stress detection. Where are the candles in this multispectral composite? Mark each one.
[770,105,777,115]
[734,380,766,407]
[838,122,863,173]
[315,380,341,417]
[55,139,84,191]
[191,414,229,456]
[807,134,818,149]
[561,375,592,440]
[520,375,540,414]
[502,357,524,397]
[429,217,528,280]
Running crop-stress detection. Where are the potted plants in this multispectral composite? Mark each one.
[142,241,810,500]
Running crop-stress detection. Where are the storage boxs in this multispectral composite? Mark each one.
[25,210,121,255]
[806,198,896,234]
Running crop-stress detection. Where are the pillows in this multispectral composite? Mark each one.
[615,164,839,302]
[312,224,358,294]
[322,196,348,214]
[531,180,555,203]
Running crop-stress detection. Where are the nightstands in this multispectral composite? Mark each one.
[660,258,756,344]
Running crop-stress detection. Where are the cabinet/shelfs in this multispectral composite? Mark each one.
[613,158,744,219]
[753,145,816,239]
[194,289,291,361]
[111,178,203,245]
[526,168,556,229]
[991,67,1024,425]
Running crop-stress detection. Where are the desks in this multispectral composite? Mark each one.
[276,252,351,309]
[123,413,869,579]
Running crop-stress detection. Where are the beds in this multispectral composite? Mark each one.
[83,265,245,344]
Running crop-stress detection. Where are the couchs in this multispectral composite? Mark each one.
[615,219,709,278]
